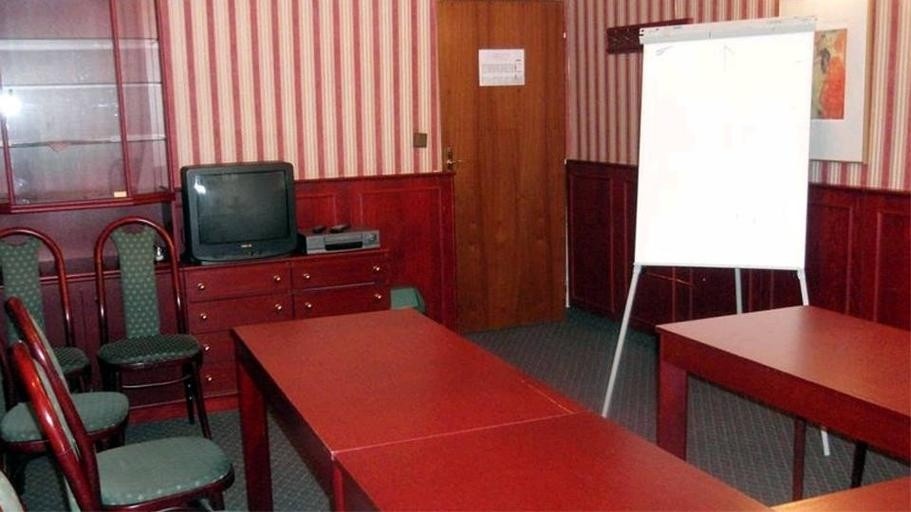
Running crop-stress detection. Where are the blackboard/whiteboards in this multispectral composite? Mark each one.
[632,32,822,268]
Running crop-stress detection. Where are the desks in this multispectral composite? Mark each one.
[335,410,775,512]
[236,307,590,510]
[653,306,910,510]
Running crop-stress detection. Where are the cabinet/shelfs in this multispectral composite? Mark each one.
[183,248,391,414]
[1,0,176,214]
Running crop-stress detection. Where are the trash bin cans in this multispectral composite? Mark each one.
[390,287,425,313]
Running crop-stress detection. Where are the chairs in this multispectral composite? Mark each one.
[0,216,234,512]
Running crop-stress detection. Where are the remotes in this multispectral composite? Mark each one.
[312,224,326,234]
[329,222,349,233]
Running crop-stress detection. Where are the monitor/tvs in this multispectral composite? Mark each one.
[180,161,298,262]
